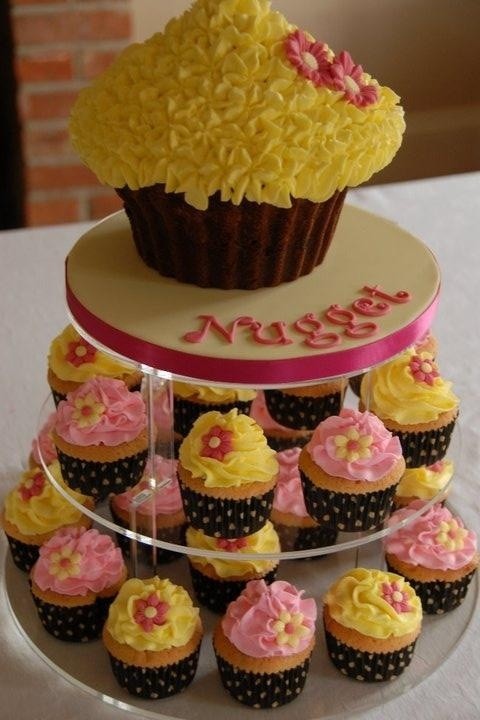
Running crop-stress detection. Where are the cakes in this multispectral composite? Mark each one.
[54,375,153,498]
[185,526,279,610]
[0,463,99,572]
[105,579,202,704]
[27,420,104,514]
[319,570,424,685]
[398,465,452,516]
[68,0,406,289]
[270,448,338,559]
[28,528,127,643]
[359,355,459,469]
[167,382,253,432]
[214,582,316,711]
[263,380,345,434]
[45,326,145,408]
[251,392,310,446]
[110,455,188,563]
[178,408,279,537]
[148,387,181,460]
[302,413,404,530]
[384,512,480,613]
